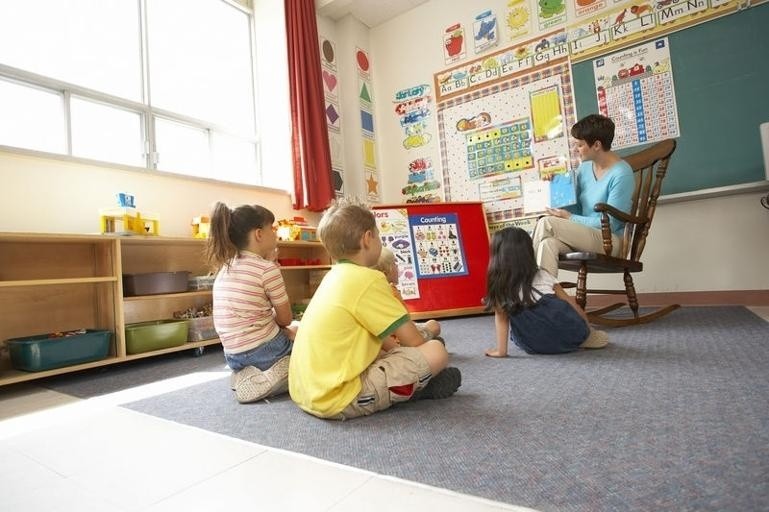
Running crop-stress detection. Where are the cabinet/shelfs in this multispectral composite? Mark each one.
[276,240,335,325]
[0,234,120,385]
[122,238,220,361]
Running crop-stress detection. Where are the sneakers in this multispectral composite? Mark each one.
[231,366,262,391]
[579,330,609,349]
[236,355,291,404]
[409,367,461,401]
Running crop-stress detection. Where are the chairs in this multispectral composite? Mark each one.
[551,139,680,329]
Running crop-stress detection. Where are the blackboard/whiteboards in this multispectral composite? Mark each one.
[568,3,769,206]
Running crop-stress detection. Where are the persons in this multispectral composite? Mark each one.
[482,227,610,357]
[287,203,462,420]
[206,201,307,403]
[368,248,445,346]
[530,114,635,279]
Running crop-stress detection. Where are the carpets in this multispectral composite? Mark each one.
[35,305,769,512]
